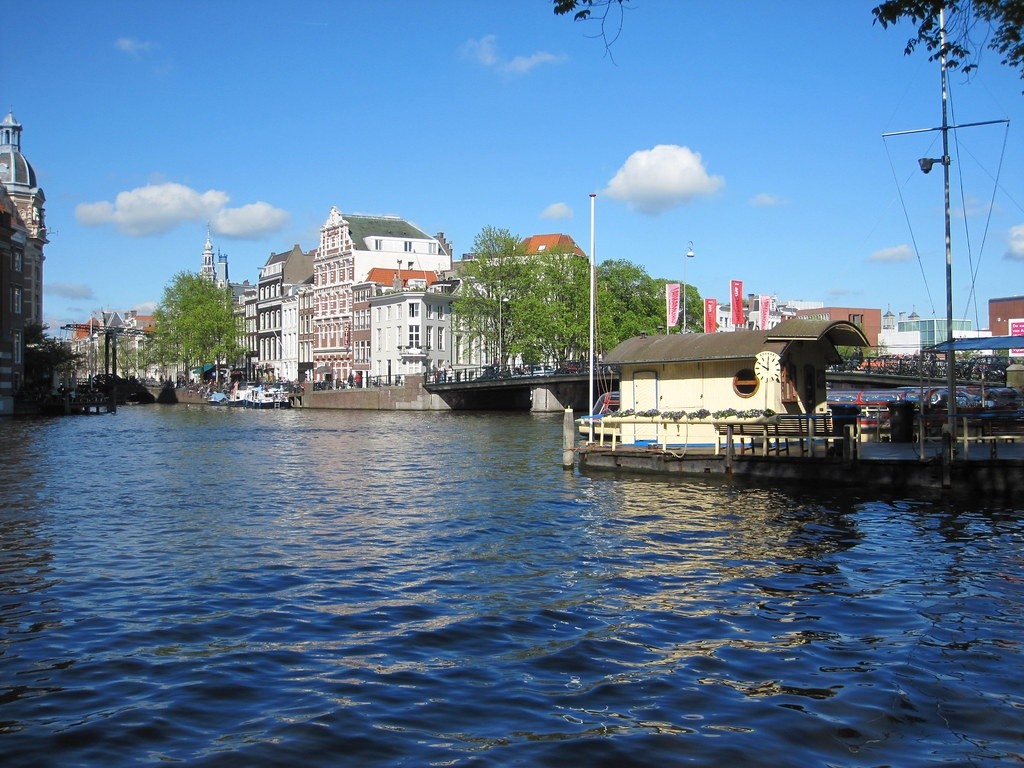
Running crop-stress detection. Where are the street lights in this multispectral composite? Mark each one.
[682,240,695,332]
[496,293,509,378]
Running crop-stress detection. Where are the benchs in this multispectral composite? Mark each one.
[925,408,1024,444]
[715,413,834,456]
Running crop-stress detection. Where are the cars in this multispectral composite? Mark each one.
[859,353,913,372]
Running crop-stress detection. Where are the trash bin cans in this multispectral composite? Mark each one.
[884,400,916,443]
[827,404,861,456]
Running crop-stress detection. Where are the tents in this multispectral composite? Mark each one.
[920,335,1024,435]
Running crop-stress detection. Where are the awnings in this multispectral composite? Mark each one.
[193,363,216,374]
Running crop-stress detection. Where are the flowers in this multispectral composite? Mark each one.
[611,407,775,420]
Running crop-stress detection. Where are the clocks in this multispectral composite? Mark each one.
[754,351,781,383]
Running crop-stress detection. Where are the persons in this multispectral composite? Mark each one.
[347,372,354,388]
[354,373,362,388]
[850,347,865,369]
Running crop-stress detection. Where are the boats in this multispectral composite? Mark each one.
[572,319,867,447]
